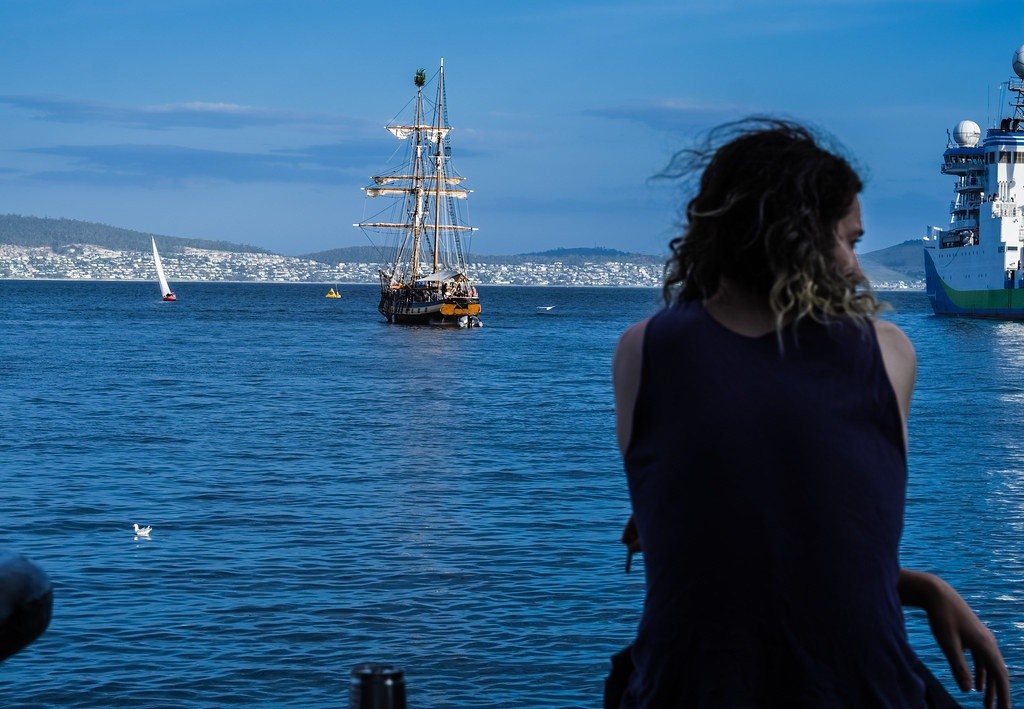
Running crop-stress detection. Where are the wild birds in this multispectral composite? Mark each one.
[536,305,558,312]
[131,523,154,536]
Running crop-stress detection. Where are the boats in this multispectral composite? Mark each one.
[923,45,1024,320]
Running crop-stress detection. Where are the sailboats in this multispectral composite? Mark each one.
[152,236,176,301]
[351,57,483,329]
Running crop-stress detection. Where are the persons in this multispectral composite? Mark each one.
[441,283,476,299]
[603,117,1009,709]
[969,230,974,246]
